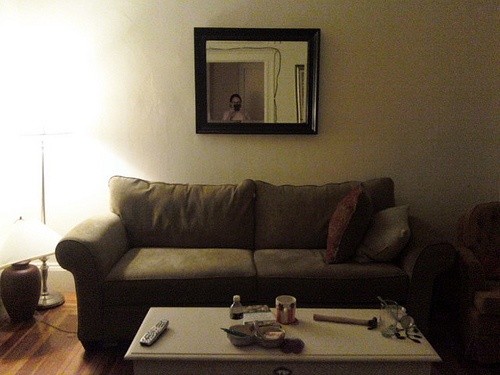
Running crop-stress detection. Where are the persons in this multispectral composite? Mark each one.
[222,93,251,122]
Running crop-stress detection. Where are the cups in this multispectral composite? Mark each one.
[380,301,398,338]
[276,295,297,324]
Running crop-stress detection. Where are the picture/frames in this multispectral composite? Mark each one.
[295,65,305,123]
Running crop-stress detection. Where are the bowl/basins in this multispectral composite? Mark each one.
[254,326,286,349]
[227,326,256,345]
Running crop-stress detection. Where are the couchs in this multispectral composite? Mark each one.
[453,201,499,365]
[55,180,456,356]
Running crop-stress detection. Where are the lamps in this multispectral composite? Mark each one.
[1,212,62,320]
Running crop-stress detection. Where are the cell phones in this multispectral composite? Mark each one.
[234,104,241,111]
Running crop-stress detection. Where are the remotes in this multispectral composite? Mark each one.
[139,319,169,346]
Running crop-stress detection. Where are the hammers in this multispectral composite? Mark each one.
[313,313,378,329]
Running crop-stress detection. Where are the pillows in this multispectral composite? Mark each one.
[324,182,373,264]
[353,204,410,264]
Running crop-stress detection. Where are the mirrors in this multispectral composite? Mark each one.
[194,27,321,135]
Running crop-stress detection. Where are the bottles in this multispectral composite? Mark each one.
[230,295,242,326]
